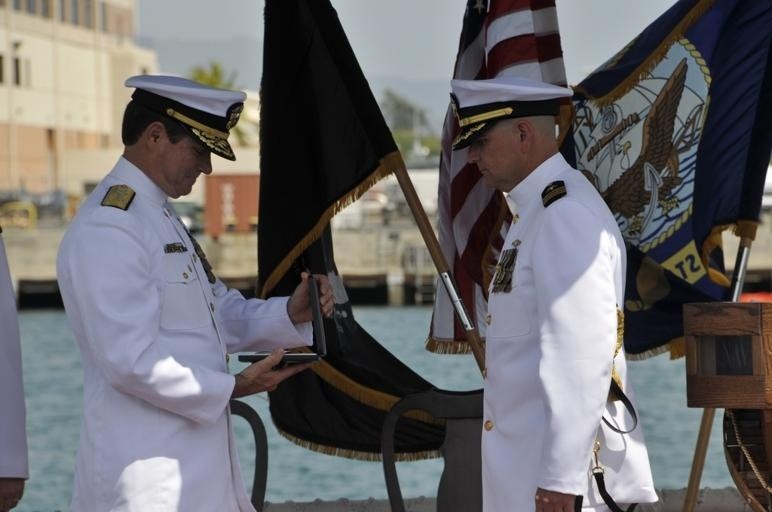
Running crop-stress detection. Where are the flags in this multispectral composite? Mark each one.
[426,0,569,354]
[560,1,772,363]
[257,1,445,463]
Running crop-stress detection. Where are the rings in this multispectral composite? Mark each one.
[12,498,21,502]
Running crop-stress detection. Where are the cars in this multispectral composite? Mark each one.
[169,202,204,233]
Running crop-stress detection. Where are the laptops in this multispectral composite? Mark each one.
[237,275,328,365]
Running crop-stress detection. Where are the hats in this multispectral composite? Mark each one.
[449,80,574,152]
[124,75,247,161]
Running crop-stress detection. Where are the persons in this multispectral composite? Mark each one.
[1,230,30,512]
[448,75,659,512]
[56,74,336,511]
